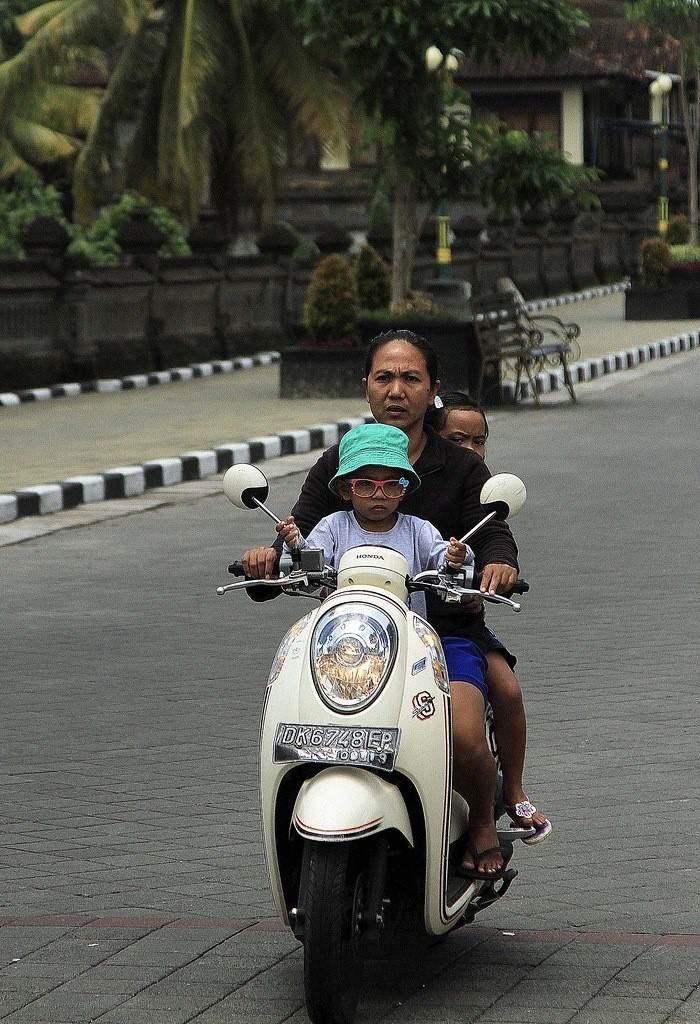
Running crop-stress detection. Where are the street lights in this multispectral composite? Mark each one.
[648,73,674,241]
[422,43,459,266]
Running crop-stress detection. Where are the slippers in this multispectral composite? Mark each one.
[457,840,504,879]
[502,792,552,844]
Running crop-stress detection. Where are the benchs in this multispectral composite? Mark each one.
[469,292,582,408]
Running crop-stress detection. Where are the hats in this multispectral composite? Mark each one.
[327,423,422,498]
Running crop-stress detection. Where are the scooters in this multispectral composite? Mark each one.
[212,461,529,1024]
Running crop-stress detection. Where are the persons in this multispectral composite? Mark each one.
[237,325,556,882]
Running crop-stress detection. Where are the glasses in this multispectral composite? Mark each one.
[344,478,410,500]
